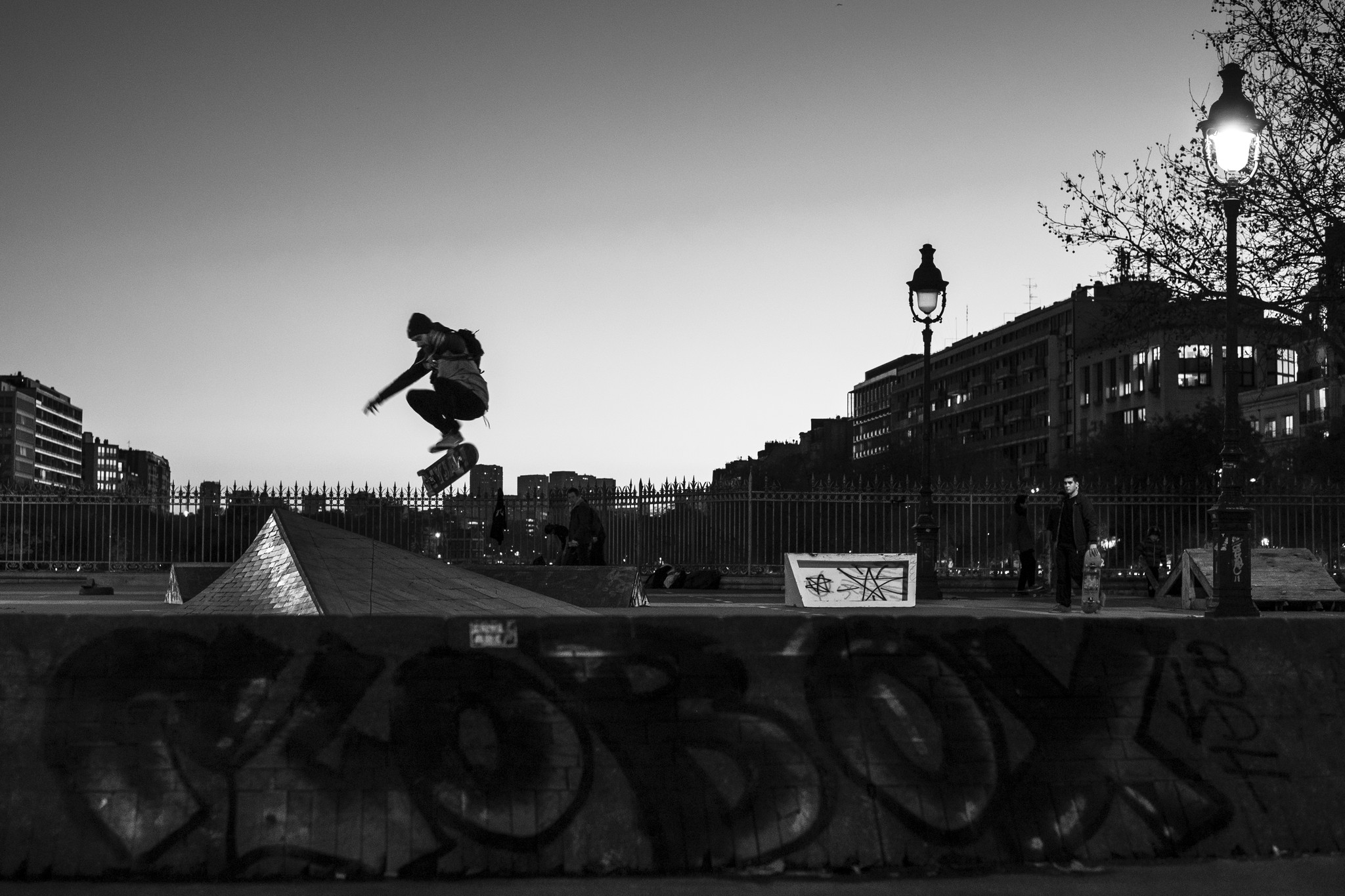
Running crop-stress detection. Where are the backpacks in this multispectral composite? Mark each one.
[645,564,721,590]
[459,329,484,368]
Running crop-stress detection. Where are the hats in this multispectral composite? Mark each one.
[407,312,433,338]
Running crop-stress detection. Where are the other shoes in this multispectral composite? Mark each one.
[1094,592,1107,614]
[1028,584,1043,591]
[436,432,464,448]
[430,442,460,453]
[1049,604,1071,614]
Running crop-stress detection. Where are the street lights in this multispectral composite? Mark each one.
[906,243,950,601]
[1197,60,1268,617]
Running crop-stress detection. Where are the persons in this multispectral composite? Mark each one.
[1051,474,1106,615]
[1142,530,1167,597]
[363,312,489,453]
[914,485,941,599]
[1012,496,1038,590]
[560,488,605,566]
[545,524,569,565]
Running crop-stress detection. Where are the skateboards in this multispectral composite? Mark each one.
[1081,549,1102,614]
[1011,586,1042,597]
[417,442,479,497]
